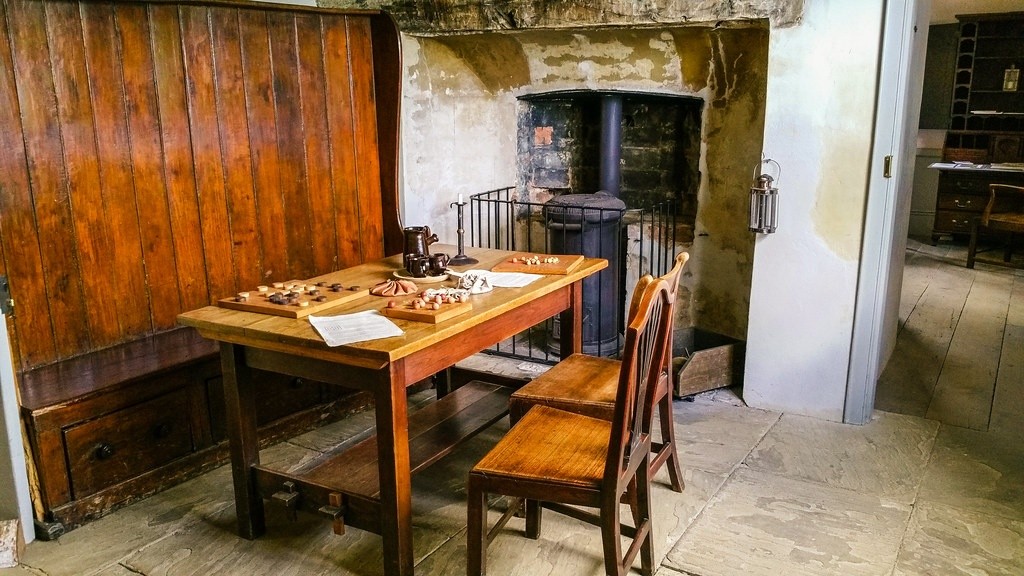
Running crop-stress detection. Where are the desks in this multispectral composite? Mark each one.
[175,244,611,576]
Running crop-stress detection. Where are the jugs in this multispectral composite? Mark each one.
[403,226,439,269]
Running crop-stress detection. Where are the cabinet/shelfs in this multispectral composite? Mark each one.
[932,170,1024,246]
[944,10,1024,163]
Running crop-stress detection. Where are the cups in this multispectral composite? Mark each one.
[406,253,451,278]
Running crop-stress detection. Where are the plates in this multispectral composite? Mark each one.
[393,268,454,283]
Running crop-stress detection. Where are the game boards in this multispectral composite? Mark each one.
[382,298,473,324]
[491,252,585,275]
[216,278,370,319]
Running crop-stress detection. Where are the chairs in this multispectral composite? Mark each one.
[469,278,675,576]
[509,252,690,494]
[967,184,1024,267]
